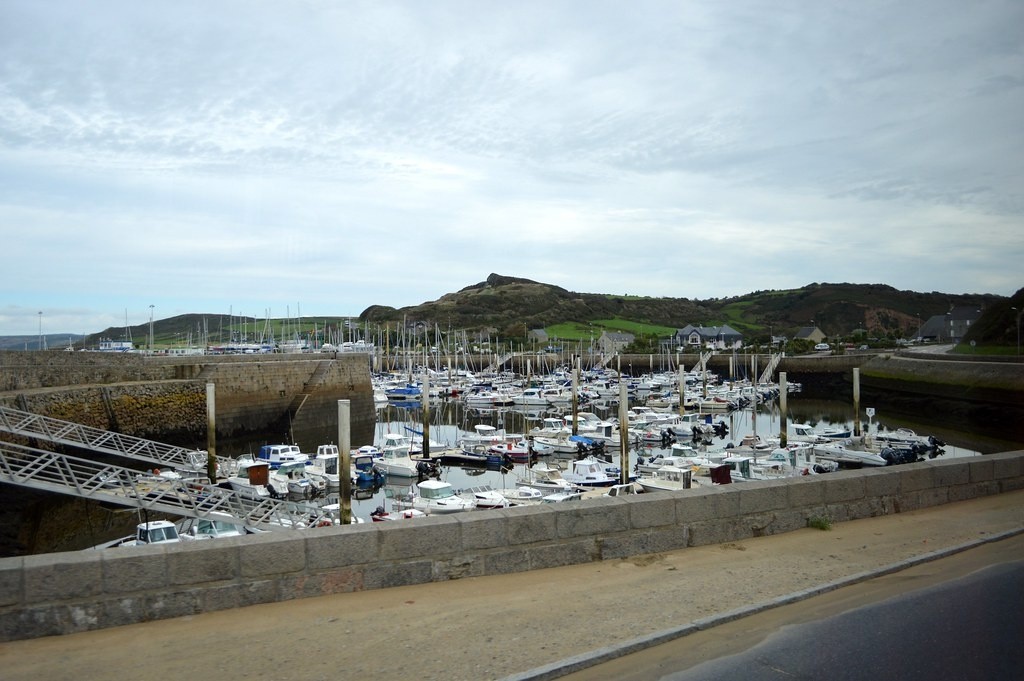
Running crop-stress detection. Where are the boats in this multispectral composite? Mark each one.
[55,302,943,553]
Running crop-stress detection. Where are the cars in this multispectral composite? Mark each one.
[814,344,830,352]
[839,341,857,348]
[860,344,869,351]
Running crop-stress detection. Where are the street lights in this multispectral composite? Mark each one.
[916,312,921,342]
[1012,306,1024,359]
[859,320,863,345]
[770,325,773,349]
[810,319,816,345]
